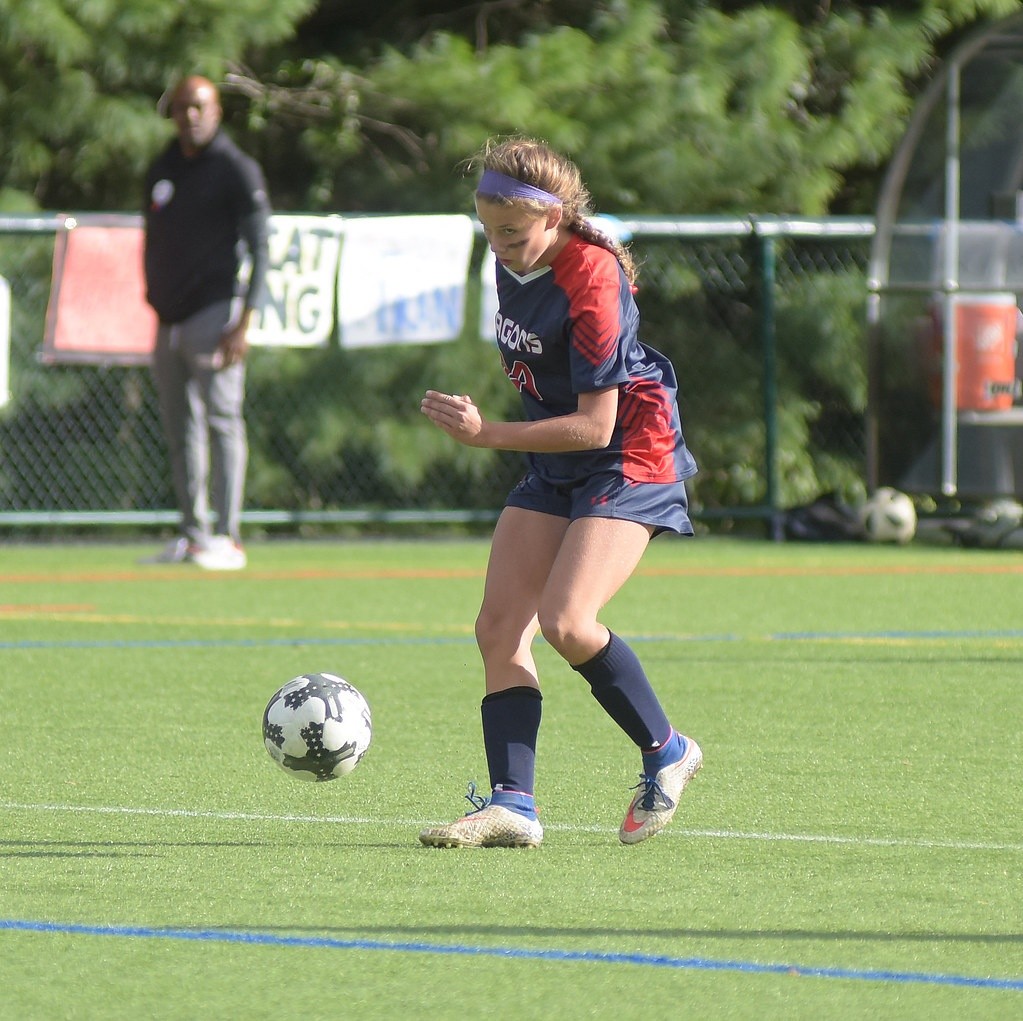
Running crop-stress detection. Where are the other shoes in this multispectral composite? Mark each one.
[193,534,245,570]
[153,536,200,561]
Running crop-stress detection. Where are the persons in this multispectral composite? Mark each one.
[419,139,702,850]
[142,74,271,569]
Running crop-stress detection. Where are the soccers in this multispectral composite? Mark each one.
[260,665,372,784]
[859,487,917,545]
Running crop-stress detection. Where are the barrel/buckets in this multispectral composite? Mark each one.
[929,292,1017,410]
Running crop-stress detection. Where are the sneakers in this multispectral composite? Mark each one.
[618,733,703,844]
[418,781,544,849]
